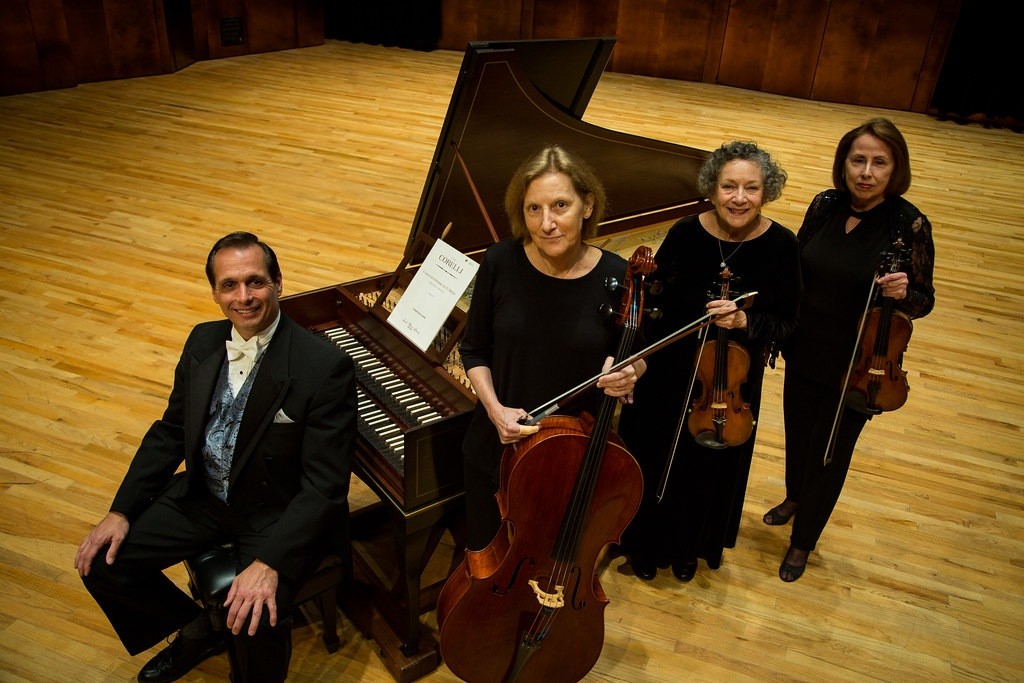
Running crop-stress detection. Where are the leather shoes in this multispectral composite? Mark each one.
[138,625,228,683]
[672,554,698,581]
[630,553,657,580]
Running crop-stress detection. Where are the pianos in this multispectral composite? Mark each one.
[267,282,492,682]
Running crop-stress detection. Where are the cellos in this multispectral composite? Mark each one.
[434,239,660,682]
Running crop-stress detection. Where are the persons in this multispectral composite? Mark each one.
[765,116,936,586]
[615,142,804,581]
[73,229,358,683]
[443,146,649,574]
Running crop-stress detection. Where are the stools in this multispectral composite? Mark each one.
[181,538,342,682]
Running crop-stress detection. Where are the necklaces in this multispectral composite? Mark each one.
[714,220,755,267]
[540,247,581,277]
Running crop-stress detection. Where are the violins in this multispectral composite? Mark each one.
[837,236,915,417]
[685,263,758,451]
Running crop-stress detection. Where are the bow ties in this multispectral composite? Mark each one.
[225,317,277,361]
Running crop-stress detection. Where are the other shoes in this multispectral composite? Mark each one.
[763,498,798,526]
[780,542,810,582]
